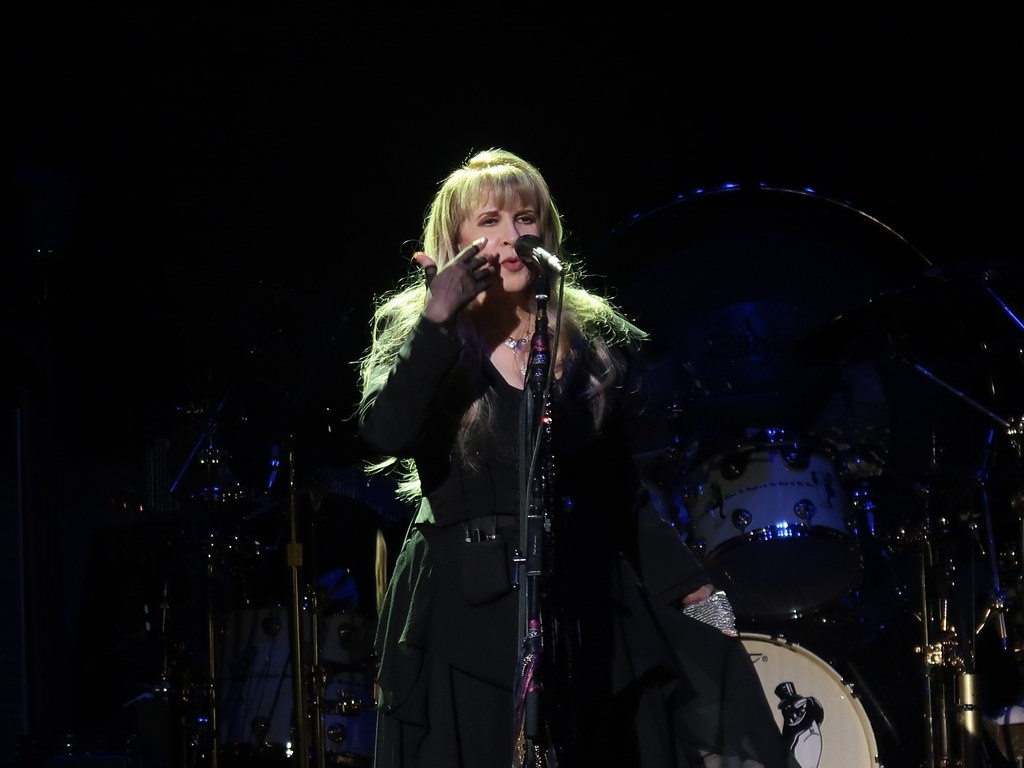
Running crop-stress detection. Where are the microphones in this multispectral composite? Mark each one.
[514,234,565,276]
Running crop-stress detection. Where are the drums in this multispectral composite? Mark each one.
[682,428,869,619]
[735,630,888,768]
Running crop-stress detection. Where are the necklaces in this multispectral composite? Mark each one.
[479,310,531,380]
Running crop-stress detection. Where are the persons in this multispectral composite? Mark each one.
[352,146,803,768]
